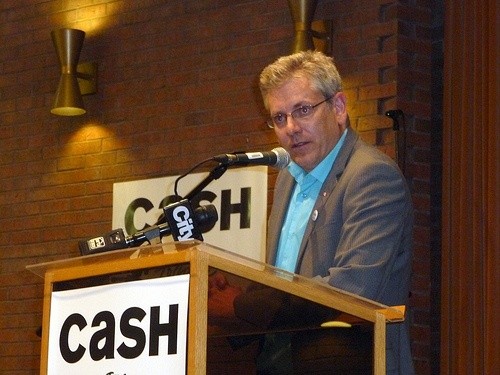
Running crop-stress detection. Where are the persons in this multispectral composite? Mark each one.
[206,49,416,375]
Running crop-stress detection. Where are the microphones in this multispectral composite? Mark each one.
[214,147,291,170]
[122,198,218,245]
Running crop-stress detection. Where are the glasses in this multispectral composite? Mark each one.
[265,97,332,128]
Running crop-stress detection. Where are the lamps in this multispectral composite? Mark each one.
[288,0,333,56]
[50,27,99,116]
